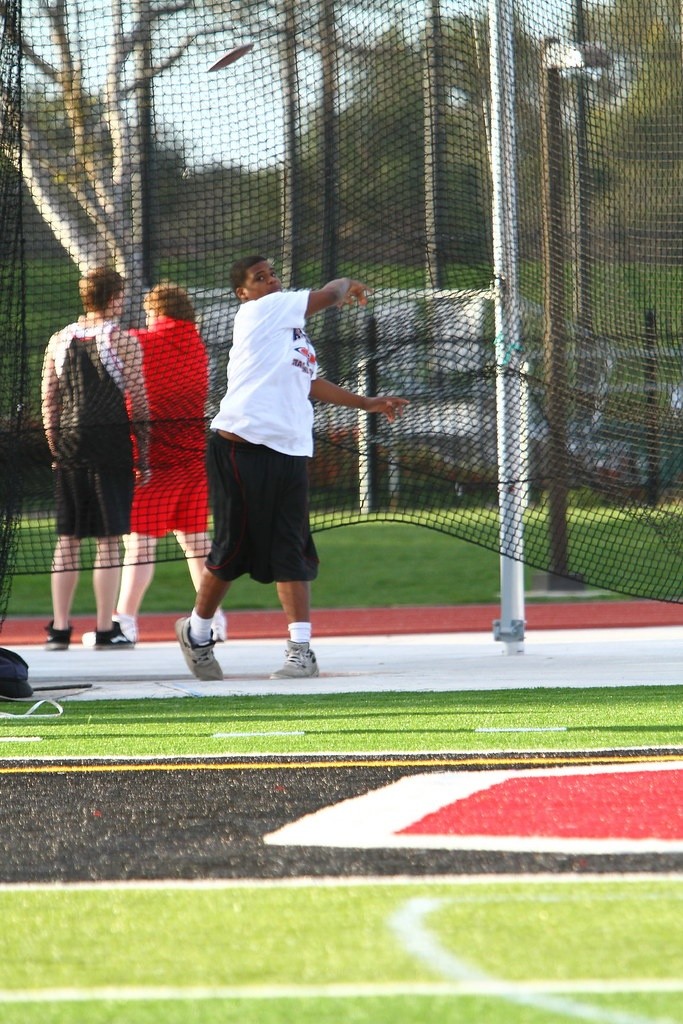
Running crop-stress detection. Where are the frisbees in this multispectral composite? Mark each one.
[204,42,255,73]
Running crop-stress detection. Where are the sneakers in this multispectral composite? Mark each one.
[174,617,224,681]
[270,639,320,681]
[46,621,74,650]
[211,609,227,643]
[82,616,138,647]
[93,621,137,649]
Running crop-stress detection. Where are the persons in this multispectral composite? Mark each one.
[40,266,150,650]
[173,255,410,679]
[83,284,225,643]
